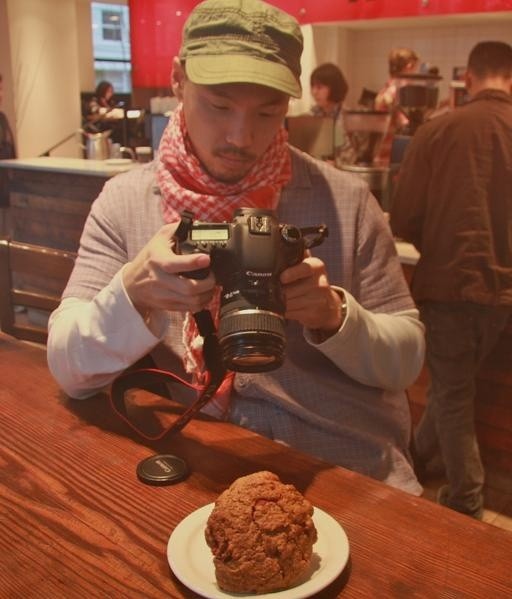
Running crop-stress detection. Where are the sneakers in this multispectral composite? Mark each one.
[437,485,484,521]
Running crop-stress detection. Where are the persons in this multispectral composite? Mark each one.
[88,81,112,117]
[388,41,511,519]
[374,47,440,110]
[307,63,369,168]
[47,0,428,499]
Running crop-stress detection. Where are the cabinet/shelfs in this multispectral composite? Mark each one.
[392,239,512,492]
[1,154,146,253]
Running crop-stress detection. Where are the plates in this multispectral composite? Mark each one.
[105,159,132,165]
[166,502,350,599]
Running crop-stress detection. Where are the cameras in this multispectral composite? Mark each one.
[171,208,328,374]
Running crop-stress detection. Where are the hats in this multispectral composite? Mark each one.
[179,0,304,99]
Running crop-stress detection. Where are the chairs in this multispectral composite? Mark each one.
[0,238,76,345]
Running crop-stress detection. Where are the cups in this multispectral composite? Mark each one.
[136,146,152,164]
